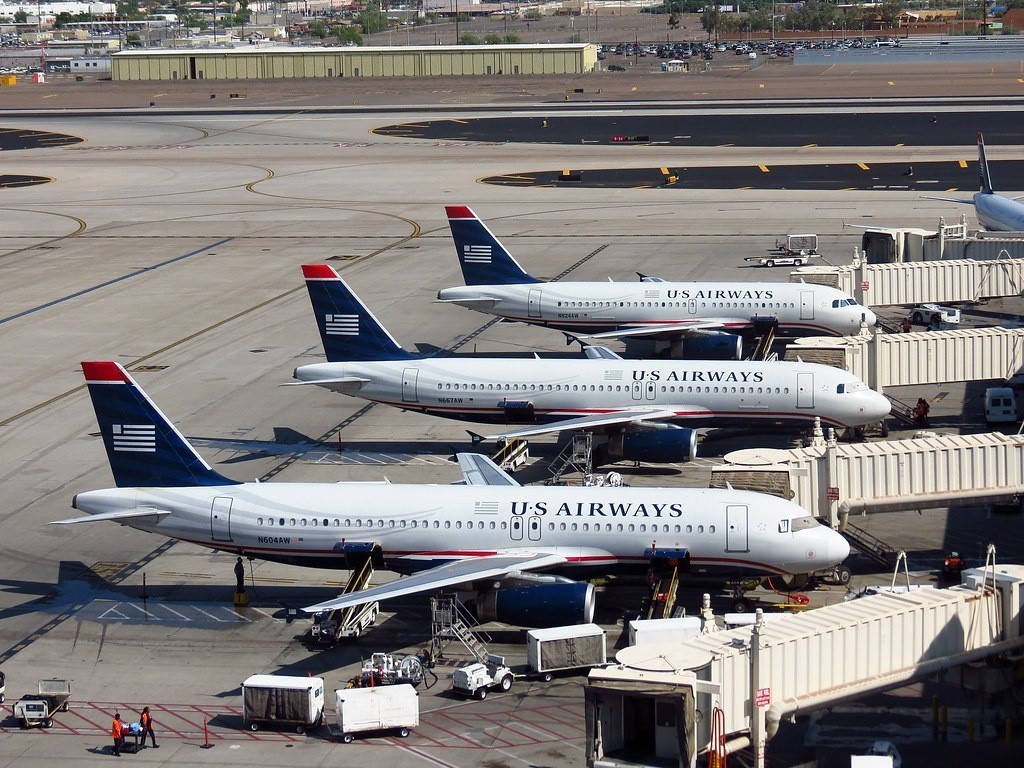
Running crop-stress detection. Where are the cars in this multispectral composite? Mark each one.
[595,36,904,73]
[0,7,417,79]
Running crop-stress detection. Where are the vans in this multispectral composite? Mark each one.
[981,387,1019,427]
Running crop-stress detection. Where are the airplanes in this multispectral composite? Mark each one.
[430,204,878,361]
[919,131,1024,233]
[46,362,853,645]
[277,264,893,470]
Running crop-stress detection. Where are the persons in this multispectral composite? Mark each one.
[234,557,245,593]
[112,714,126,757]
[899,318,912,333]
[423,649,430,659]
[911,398,931,420]
[140,706,160,749]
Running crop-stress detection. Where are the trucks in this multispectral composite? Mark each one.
[452,623,607,701]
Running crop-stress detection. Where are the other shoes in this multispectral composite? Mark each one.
[153,743,160,748]
[114,754,120,756]
[142,745,147,748]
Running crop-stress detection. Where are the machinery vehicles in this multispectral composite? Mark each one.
[907,302,960,328]
[743,233,824,269]
